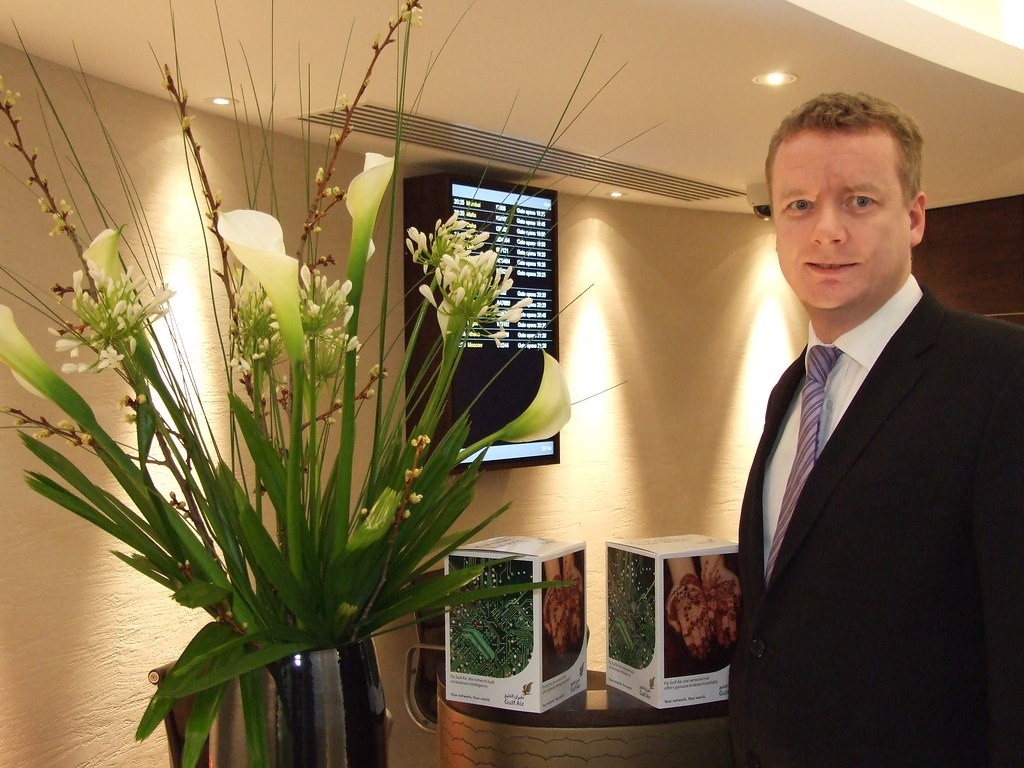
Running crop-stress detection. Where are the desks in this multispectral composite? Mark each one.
[436,663,736,768]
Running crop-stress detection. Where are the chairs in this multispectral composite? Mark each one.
[402,569,446,734]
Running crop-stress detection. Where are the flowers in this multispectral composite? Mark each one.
[0,1,670,768]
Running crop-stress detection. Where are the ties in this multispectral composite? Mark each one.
[764,345,845,591]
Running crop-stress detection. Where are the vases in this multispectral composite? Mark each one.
[206,633,389,768]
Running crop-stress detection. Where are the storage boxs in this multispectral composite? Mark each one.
[606,533,738,709]
[444,536,588,714]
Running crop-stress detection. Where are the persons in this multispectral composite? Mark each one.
[727,92,1023,767]
